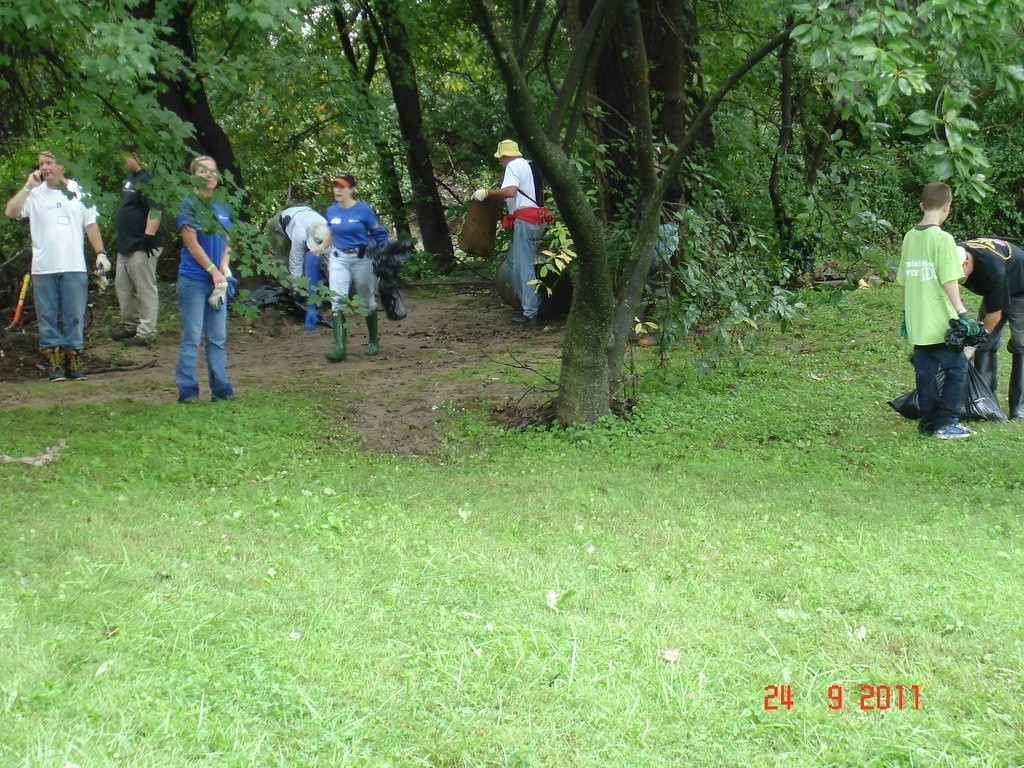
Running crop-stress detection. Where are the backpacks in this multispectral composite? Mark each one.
[503,157,545,207]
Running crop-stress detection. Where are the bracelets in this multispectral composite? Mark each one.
[319,245,326,251]
[23,186,31,194]
[96,250,107,254]
[486,189,489,195]
[207,263,216,274]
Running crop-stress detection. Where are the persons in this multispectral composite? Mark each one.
[628,145,680,346]
[6,151,111,382]
[955,237,1024,421]
[111,142,167,347]
[175,156,233,406]
[896,182,980,441]
[471,140,542,323]
[307,173,387,362]
[268,206,332,330]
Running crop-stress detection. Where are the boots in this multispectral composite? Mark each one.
[42,346,66,382]
[364,311,378,356]
[63,350,87,380]
[327,310,346,363]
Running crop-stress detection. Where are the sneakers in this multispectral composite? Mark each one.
[112,326,136,341]
[511,312,535,323]
[934,423,977,440]
[123,336,146,346]
[920,429,935,439]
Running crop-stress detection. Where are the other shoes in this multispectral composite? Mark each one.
[178,398,200,405]
[212,395,241,402]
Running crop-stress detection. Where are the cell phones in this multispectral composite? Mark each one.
[40,174,44,181]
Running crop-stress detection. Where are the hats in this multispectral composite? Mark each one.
[957,246,967,266]
[330,173,355,187]
[306,223,331,254]
[494,139,522,158]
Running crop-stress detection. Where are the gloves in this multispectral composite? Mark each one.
[208,282,229,310]
[97,253,111,273]
[141,234,160,258]
[900,311,907,339]
[471,187,488,201]
[226,275,238,299]
[958,311,980,336]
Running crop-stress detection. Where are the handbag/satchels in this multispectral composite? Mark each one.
[372,236,415,319]
[885,317,1009,423]
